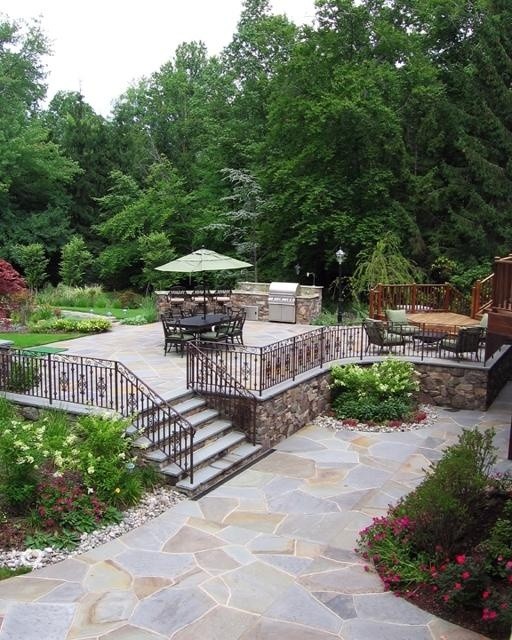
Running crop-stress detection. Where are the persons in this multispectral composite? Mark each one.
[294,262,302,282]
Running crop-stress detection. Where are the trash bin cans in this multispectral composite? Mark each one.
[268,282,300,323]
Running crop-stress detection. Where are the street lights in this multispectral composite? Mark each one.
[332,245,347,327]
[304,271,316,287]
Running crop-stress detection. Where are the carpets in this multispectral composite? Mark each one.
[14,346,69,358]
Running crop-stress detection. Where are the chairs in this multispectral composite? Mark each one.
[160,304,247,358]
[362,310,488,364]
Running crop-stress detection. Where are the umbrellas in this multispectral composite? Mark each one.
[154,248,255,320]
